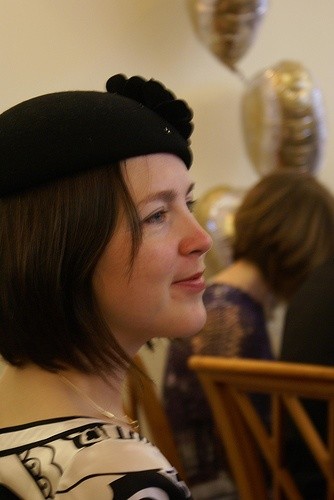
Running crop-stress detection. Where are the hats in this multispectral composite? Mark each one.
[0,75,192,200]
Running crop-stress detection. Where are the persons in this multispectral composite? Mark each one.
[0,74,212,500]
[162,172,334,500]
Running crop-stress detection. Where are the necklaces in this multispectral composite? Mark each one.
[57,374,138,427]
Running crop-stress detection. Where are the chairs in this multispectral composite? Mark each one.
[188,356,334,498]
